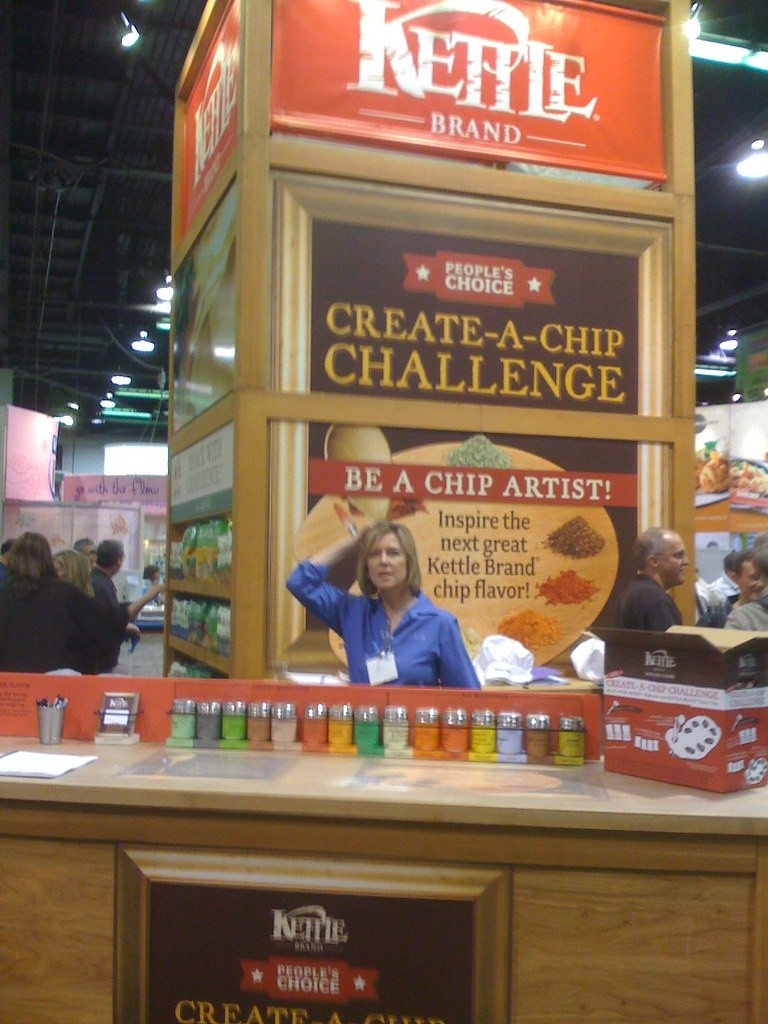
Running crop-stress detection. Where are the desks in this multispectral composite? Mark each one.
[481,674,604,694]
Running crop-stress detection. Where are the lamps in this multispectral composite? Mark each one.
[684,3,704,41]
[113,7,140,47]
[131,330,155,352]
[155,275,172,301]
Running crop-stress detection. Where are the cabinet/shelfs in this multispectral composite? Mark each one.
[167,575,234,675]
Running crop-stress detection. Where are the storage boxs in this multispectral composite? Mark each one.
[585,623,768,795]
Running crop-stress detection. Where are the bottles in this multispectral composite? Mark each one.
[168,700,585,761]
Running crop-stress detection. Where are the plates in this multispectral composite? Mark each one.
[694,454,768,509]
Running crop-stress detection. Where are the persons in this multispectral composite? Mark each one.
[285,520,481,690]
[615,527,768,632]
[0,531,166,674]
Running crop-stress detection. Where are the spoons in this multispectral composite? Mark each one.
[324,421,393,523]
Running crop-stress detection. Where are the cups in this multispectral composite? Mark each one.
[37,706,63,745]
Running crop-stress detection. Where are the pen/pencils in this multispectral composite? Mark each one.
[36,695,70,708]
